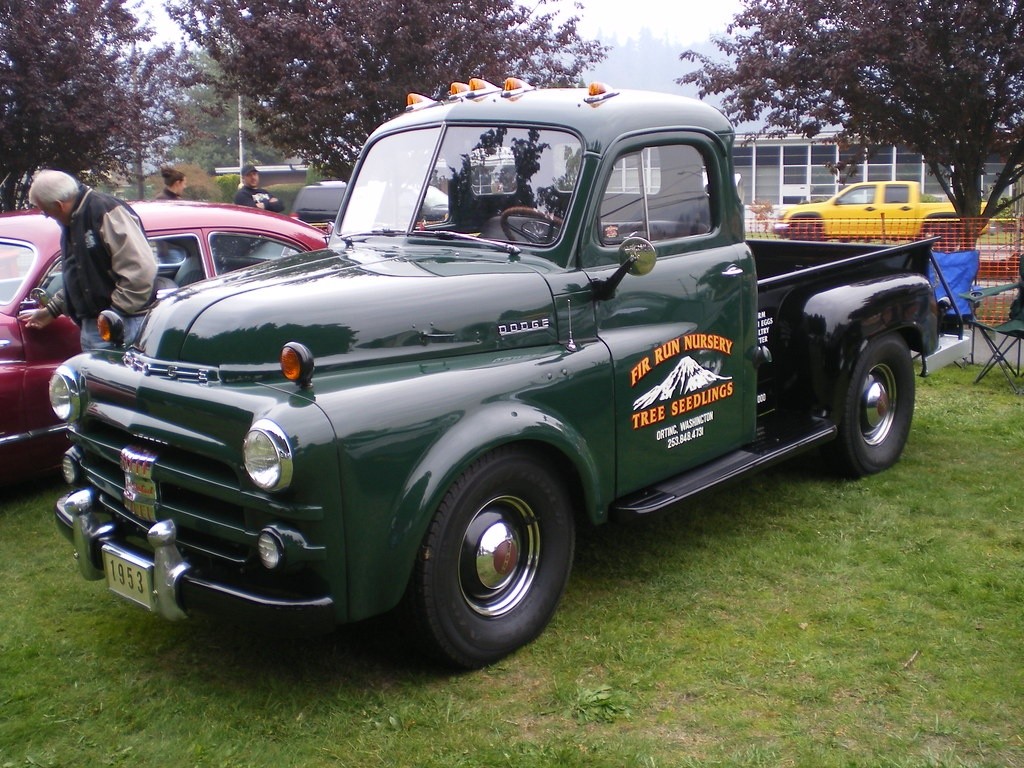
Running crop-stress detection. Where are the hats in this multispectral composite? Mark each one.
[241,164,259,176]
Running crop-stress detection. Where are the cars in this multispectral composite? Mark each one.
[0,200,331,482]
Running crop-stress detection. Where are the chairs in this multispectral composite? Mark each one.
[959,254,1024,395]
[153,256,205,293]
[911,249,982,369]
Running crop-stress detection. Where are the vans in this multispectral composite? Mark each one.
[291,180,451,231]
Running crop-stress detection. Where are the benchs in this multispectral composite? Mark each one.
[602,219,710,245]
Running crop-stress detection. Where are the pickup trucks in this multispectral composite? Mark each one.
[773,181,992,252]
[49,80,942,674]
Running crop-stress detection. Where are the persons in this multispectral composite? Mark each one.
[234,164,285,213]
[154,166,186,199]
[12,169,162,352]
